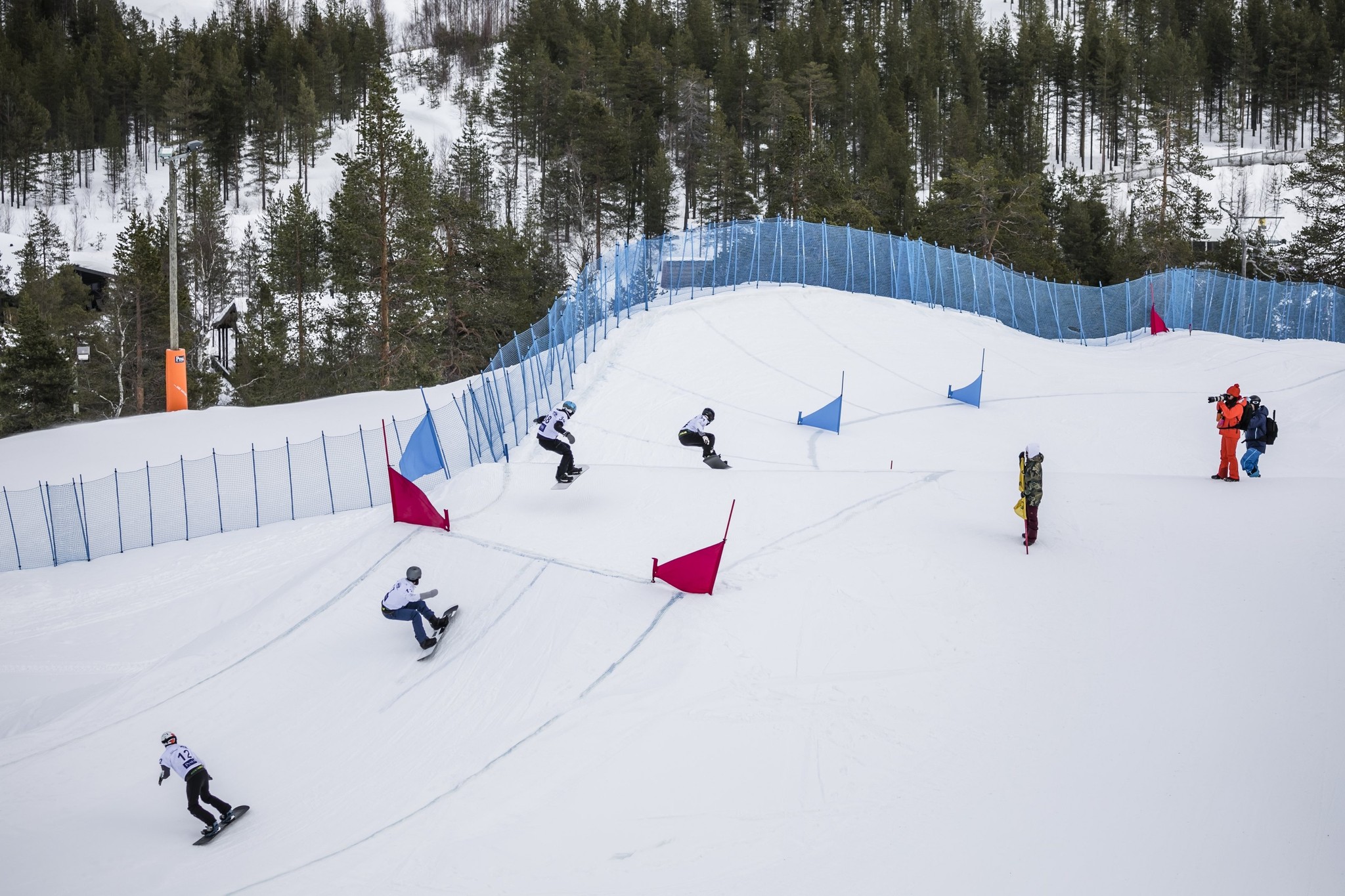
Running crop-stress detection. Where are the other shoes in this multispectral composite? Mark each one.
[1022,533,1033,546]
[1211,475,1240,482]
[429,614,449,630]
[415,635,437,650]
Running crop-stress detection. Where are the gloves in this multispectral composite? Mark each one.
[1019,452,1024,459]
[565,431,575,444]
[1021,491,1025,498]
[420,589,438,600]
[533,418,538,423]
[159,776,163,786]
[702,435,710,445]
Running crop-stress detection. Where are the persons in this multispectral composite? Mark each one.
[1019,442,1044,547]
[382,566,449,649]
[678,408,727,467]
[1211,384,1267,482]
[533,401,582,482]
[159,732,235,835]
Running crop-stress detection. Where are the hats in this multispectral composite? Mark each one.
[1026,443,1040,458]
[1227,384,1240,397]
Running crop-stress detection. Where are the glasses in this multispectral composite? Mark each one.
[1226,394,1233,398]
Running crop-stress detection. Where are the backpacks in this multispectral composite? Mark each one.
[1231,401,1253,431]
[1253,413,1278,445]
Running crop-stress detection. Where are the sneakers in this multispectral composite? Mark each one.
[555,466,573,482]
[567,465,582,475]
[219,809,235,821]
[201,821,221,837]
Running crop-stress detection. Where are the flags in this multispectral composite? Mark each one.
[1014,458,1027,520]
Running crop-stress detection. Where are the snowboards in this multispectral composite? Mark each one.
[703,455,733,469]
[417,605,458,661]
[551,464,590,490]
[192,805,250,845]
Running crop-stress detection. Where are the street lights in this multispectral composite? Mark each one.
[159,139,203,409]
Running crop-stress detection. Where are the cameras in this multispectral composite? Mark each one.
[1207,393,1228,403]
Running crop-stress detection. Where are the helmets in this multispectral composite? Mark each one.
[1249,395,1261,410]
[562,401,576,416]
[161,731,177,747]
[702,408,715,423]
[406,566,422,580]
